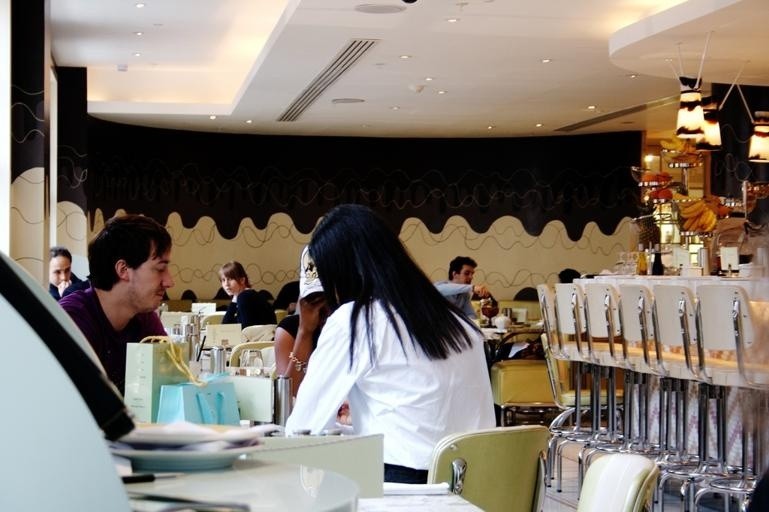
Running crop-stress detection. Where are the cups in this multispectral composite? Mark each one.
[502,307,513,323]
[201,357,211,373]
[664,234,672,243]
[495,315,512,329]
[691,236,699,243]
[188,361,201,376]
[238,349,264,379]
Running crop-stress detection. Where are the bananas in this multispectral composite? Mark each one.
[659,134,684,164]
[671,193,716,234]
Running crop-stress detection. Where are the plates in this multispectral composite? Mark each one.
[111,447,263,472]
[121,430,221,449]
[126,468,362,511]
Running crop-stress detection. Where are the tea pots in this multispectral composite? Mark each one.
[481,294,500,318]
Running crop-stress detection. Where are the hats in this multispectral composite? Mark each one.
[299,245,326,299]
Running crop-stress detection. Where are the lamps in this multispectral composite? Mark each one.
[694,59,746,151]
[666,30,712,139]
[730,77,769,164]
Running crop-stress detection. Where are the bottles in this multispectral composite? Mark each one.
[635,244,649,275]
[169,315,201,360]
[212,346,225,374]
[616,251,638,274]
[274,376,293,425]
[678,264,683,276]
[700,247,710,276]
[757,246,768,277]
[650,243,664,275]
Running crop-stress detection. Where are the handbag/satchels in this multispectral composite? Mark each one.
[124,336,189,424]
[157,379,240,427]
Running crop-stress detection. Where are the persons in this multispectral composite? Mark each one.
[273,279,301,316]
[49,246,87,299]
[53,215,174,398]
[431,255,491,328]
[273,240,351,424]
[282,203,497,484]
[216,260,277,330]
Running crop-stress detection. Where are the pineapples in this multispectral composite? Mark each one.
[638,206,660,250]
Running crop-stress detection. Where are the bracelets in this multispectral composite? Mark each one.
[472,285,475,292]
[289,352,309,376]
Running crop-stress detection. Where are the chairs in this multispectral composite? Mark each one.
[151,296,298,426]
[425,423,551,512]
[467,287,562,432]
[534,278,763,511]
